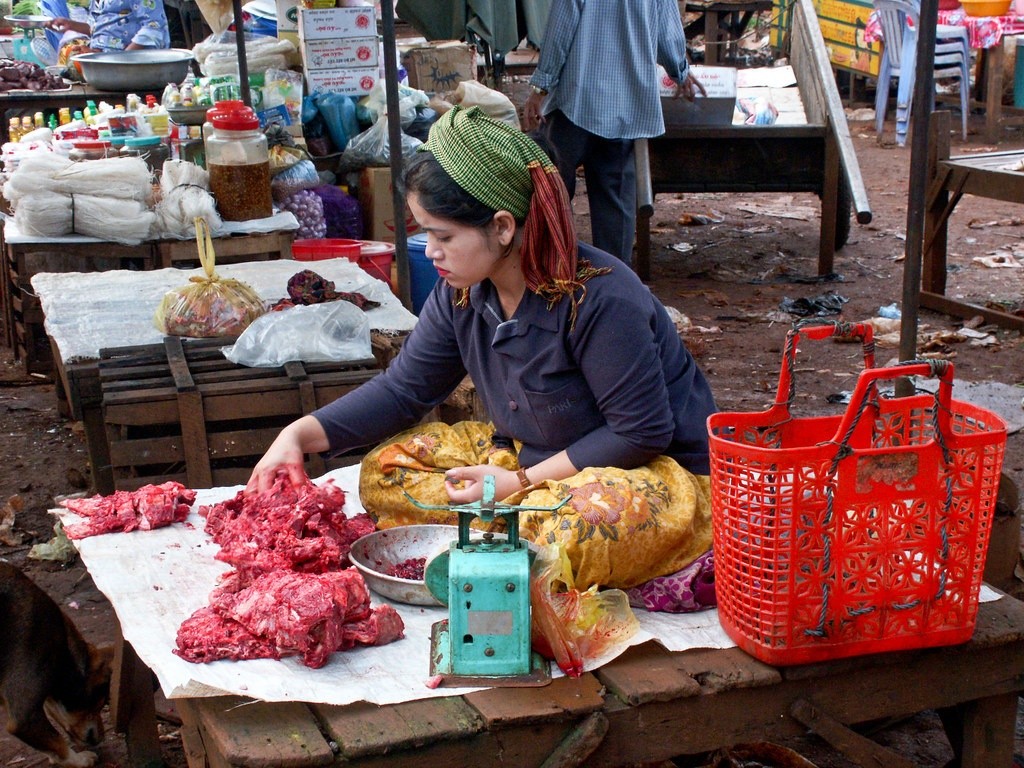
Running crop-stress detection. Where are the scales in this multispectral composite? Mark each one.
[404,476,573,688]
[167,108,206,168]
[3,15,60,69]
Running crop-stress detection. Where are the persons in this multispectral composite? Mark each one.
[243,108,728,591]
[522,0,706,265]
[41,0,169,79]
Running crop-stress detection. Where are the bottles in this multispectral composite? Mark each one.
[202,100,273,222]
[7,81,194,188]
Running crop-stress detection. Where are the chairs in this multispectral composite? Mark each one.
[871,0,971,147]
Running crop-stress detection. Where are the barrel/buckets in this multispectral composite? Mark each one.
[350,239,396,290]
[407,233,440,317]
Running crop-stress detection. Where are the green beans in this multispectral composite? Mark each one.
[10,0,43,17]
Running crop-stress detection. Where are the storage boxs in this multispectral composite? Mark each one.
[298,40,379,68]
[655,65,737,126]
[275,1,302,29]
[958,0,1012,17]
[290,239,364,271]
[296,5,379,40]
[938,0,961,11]
[303,69,379,97]
[277,31,300,48]
[360,168,420,246]
[406,47,477,92]
[408,234,441,314]
[1015,39,1024,112]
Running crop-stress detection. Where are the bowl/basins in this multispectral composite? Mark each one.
[959,0,1012,16]
[348,524,552,606]
[71,48,195,91]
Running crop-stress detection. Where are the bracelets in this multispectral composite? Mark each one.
[516,466,531,488]
[533,87,547,96]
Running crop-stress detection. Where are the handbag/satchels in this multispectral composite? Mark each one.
[155,217,269,339]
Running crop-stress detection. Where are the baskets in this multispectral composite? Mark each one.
[707,322,1009,667]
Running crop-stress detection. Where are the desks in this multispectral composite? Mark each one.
[477,51,540,92]
[936,12,1024,144]
[635,83,839,283]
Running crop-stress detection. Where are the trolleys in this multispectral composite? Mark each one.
[632,1,871,283]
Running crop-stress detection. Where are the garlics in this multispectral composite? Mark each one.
[277,190,327,241]
